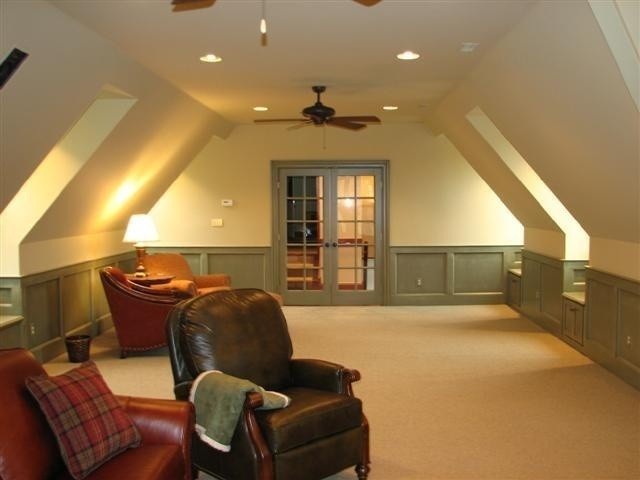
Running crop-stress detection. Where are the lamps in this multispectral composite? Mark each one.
[123,214,160,277]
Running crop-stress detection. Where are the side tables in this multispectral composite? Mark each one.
[124,274,176,287]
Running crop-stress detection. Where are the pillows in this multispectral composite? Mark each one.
[26,361,143,480]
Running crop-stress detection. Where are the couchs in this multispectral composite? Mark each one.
[0,348,195,480]
[146,253,231,299]
[166,288,371,480]
[99,266,193,359]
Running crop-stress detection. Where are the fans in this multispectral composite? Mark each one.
[253,85,381,133]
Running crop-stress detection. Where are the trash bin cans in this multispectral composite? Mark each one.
[66,335,93,363]
